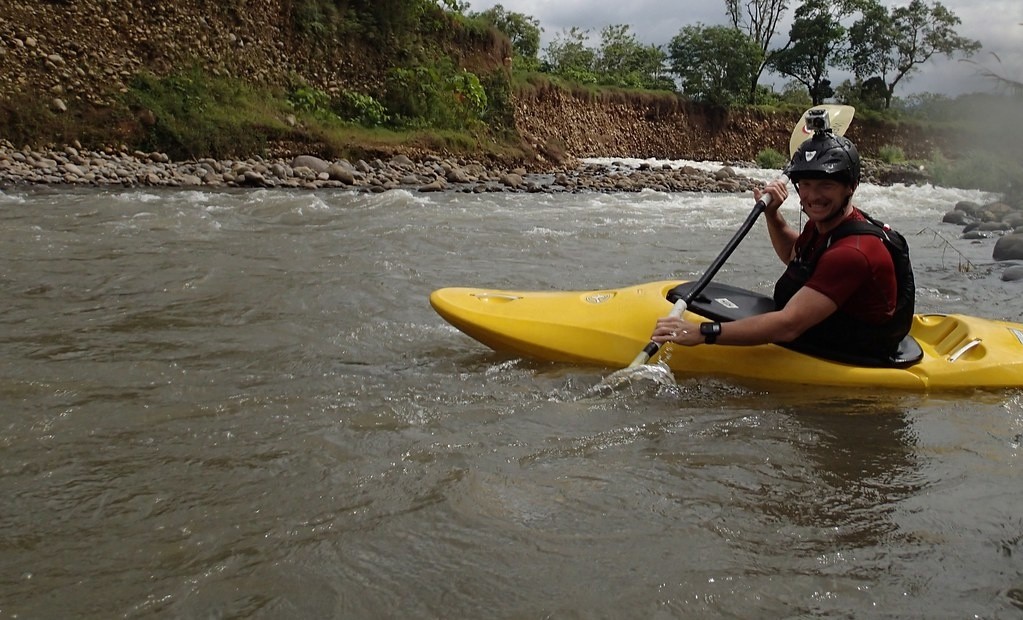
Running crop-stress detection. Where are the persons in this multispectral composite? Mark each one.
[650,134,916,367]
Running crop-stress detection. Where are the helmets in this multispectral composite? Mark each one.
[783,134,861,191]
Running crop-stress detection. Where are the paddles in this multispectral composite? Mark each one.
[625,104,855,370]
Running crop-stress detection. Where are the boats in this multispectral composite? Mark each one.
[428,279,1023,390]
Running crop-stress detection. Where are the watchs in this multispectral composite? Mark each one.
[700,320,721,344]
